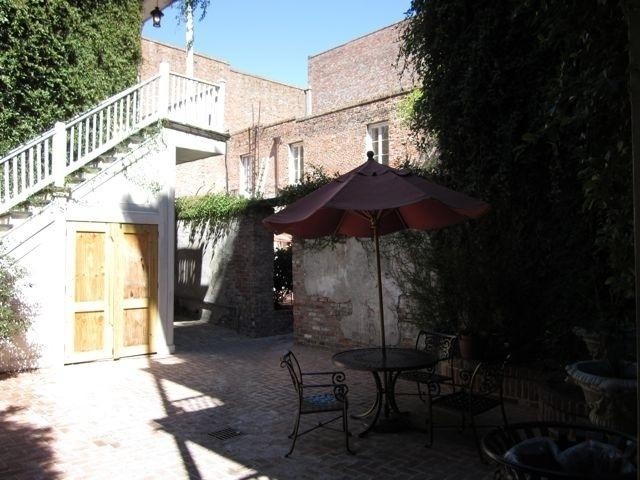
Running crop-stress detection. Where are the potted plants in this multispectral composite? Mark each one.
[558,129,639,436]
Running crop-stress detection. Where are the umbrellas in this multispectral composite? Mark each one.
[262,151,492,417]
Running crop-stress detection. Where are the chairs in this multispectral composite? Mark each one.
[278,330,515,466]
